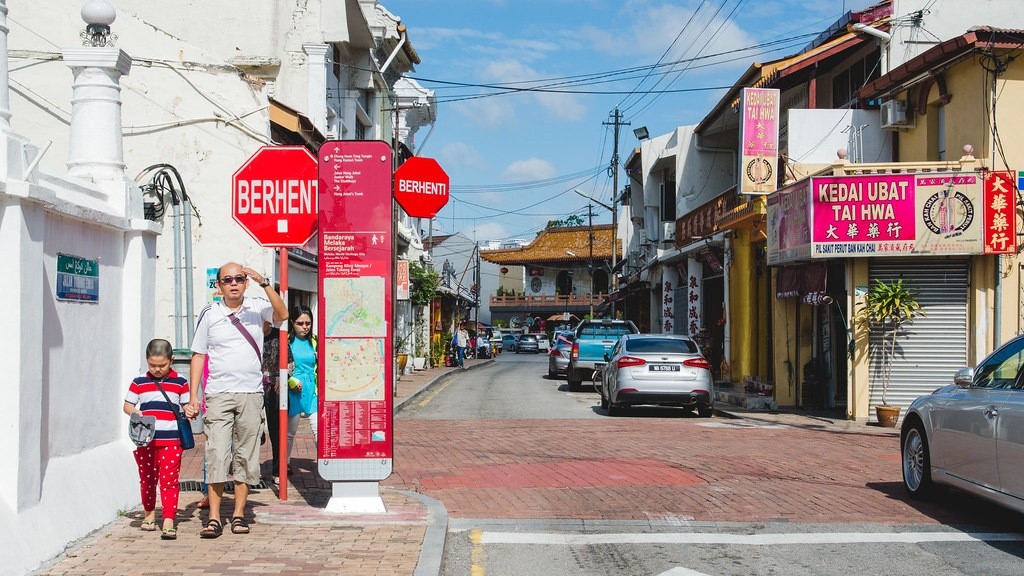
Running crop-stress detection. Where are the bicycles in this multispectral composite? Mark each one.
[592,362,605,397]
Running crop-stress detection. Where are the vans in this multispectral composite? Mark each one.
[486,330,503,353]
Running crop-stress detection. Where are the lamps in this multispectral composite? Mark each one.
[786,171,796,180]
[837,149,847,159]
[79,0,120,48]
[633,125,651,142]
[963,145,974,155]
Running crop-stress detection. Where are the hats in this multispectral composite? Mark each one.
[128,413,156,446]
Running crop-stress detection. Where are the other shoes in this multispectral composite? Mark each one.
[288,465,293,476]
[198,496,210,508]
[271,473,281,484]
[457,365,463,368]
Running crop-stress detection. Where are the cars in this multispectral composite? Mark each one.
[502,334,521,352]
[515,334,539,354]
[547,333,575,379]
[600,332,714,418]
[533,333,551,352]
[553,330,571,345]
[899,333,1024,521]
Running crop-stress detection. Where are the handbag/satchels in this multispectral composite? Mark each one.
[452,330,458,346]
[177,418,195,450]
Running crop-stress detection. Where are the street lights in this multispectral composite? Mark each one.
[566,251,594,320]
[573,187,618,320]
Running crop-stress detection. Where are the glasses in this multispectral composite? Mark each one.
[293,320,311,326]
[219,276,246,285]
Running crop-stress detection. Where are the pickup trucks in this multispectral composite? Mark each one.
[566,320,641,392]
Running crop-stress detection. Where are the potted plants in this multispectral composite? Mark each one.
[396,259,453,375]
[850,272,926,428]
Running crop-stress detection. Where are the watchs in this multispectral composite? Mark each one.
[260,278,270,287]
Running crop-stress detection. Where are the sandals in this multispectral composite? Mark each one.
[200,519,223,537]
[230,516,249,533]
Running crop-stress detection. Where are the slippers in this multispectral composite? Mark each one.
[161,527,177,539]
[141,518,156,531]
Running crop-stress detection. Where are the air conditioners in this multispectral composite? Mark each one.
[662,222,675,241]
[880,99,907,127]
[638,229,646,245]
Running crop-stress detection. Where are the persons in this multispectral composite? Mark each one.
[187,263,290,540]
[470,335,475,348]
[123,339,199,541]
[288,303,317,478]
[477,334,486,359]
[449,323,472,368]
[261,321,294,484]
